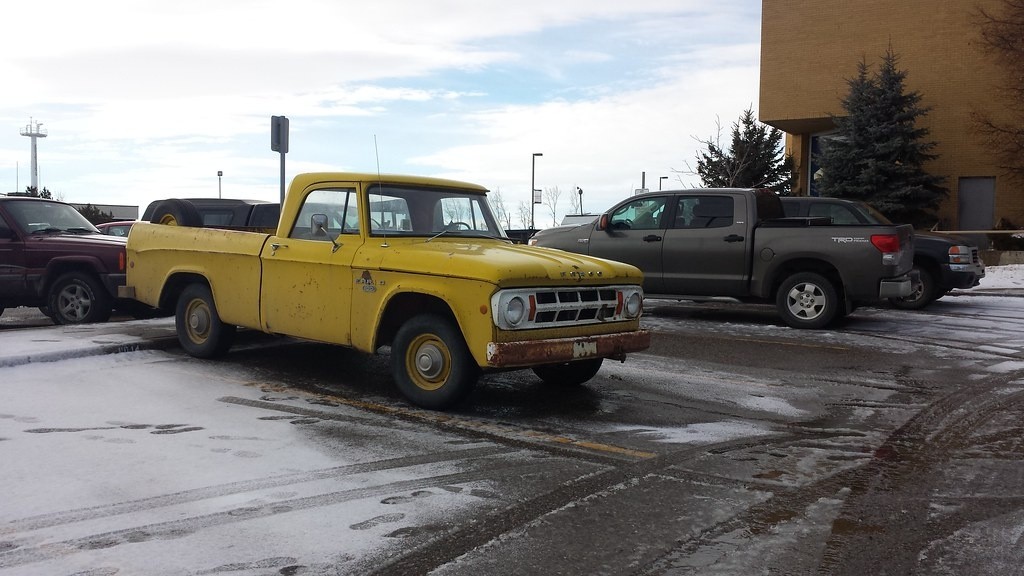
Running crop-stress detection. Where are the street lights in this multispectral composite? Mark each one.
[531,152,543,229]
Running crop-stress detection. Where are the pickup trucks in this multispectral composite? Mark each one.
[0,171,651,412]
[528,187,986,329]
